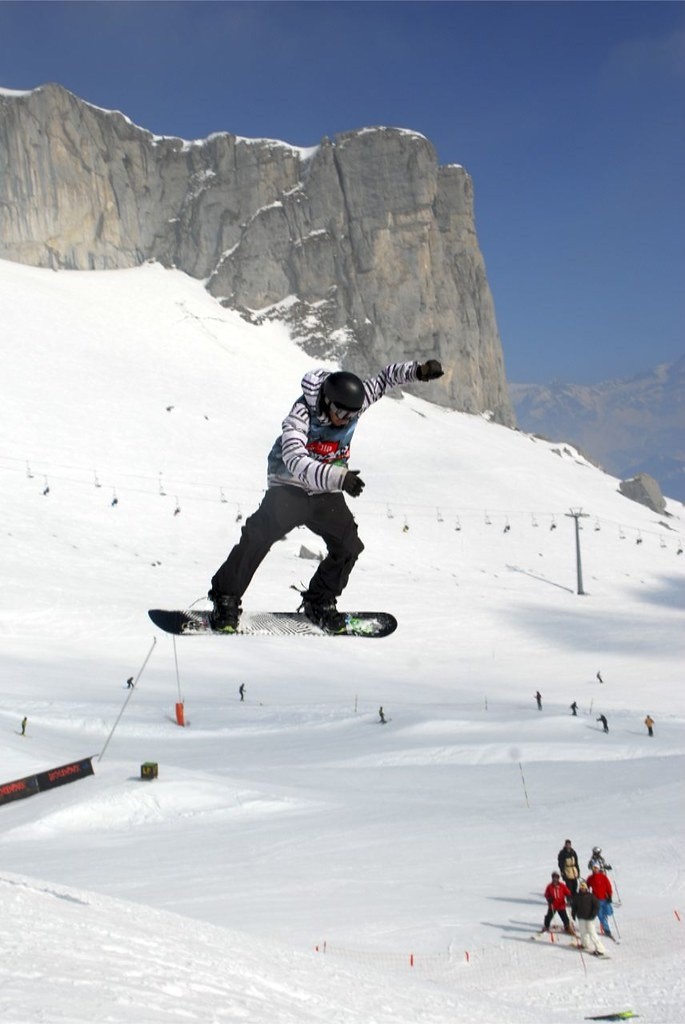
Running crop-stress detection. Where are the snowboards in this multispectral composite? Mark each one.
[149,608,397,639]
[571,942,611,960]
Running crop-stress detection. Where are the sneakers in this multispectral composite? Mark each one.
[293,598,346,625]
[208,588,243,633]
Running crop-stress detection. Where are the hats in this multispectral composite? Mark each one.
[593,863,600,870]
[580,883,587,888]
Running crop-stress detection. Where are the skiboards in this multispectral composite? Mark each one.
[530,931,582,942]
[609,934,624,946]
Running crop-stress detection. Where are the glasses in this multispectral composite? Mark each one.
[324,397,359,420]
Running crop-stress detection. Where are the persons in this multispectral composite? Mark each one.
[379,706,387,723]
[541,839,613,955]
[534,691,542,710]
[644,715,654,737]
[207,359,444,633]
[570,701,578,716]
[127,677,134,689]
[21,717,27,735]
[239,683,246,702]
[596,714,609,734]
[596,671,604,683]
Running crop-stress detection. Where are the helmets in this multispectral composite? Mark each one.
[325,371,365,411]
[593,847,601,855]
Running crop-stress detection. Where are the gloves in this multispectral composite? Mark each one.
[607,896,612,903]
[416,360,444,382]
[343,471,365,497]
